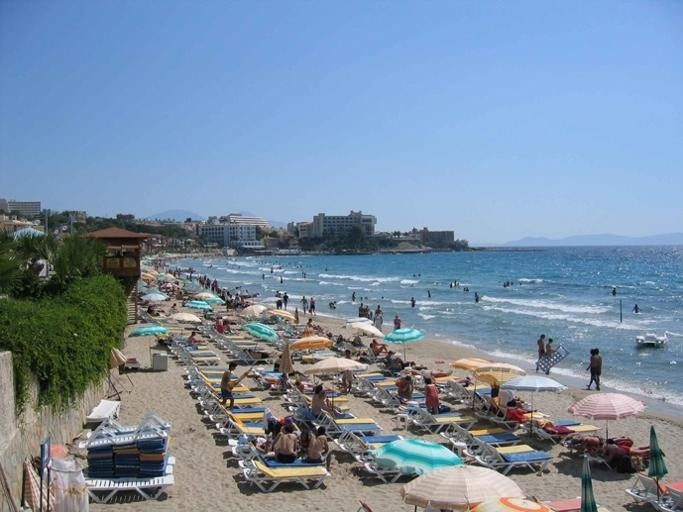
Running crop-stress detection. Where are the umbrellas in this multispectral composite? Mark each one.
[649,424,668,501]
[128,325,167,365]
[580,454,600,512]
[399,464,525,511]
[23,457,55,512]
[111,347,134,386]
[464,496,555,512]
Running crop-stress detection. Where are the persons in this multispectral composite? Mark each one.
[136,255,666,464]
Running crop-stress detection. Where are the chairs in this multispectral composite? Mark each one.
[77,422,169,449]
[85,414,172,437]
[81,477,175,503]
